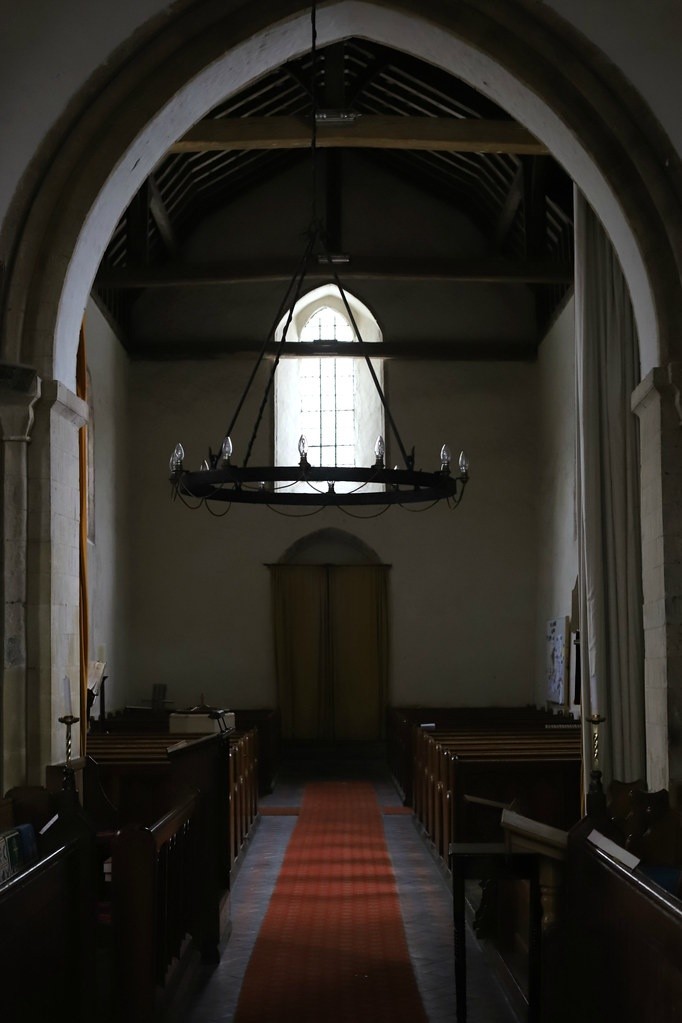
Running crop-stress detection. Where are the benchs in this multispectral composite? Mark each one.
[388,705,583,877]
[87,709,275,889]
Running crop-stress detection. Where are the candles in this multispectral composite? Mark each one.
[591,677,599,714]
[62,677,73,717]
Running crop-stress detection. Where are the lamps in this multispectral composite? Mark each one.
[170,1,468,518]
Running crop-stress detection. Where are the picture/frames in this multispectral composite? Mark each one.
[546,616,569,703]
